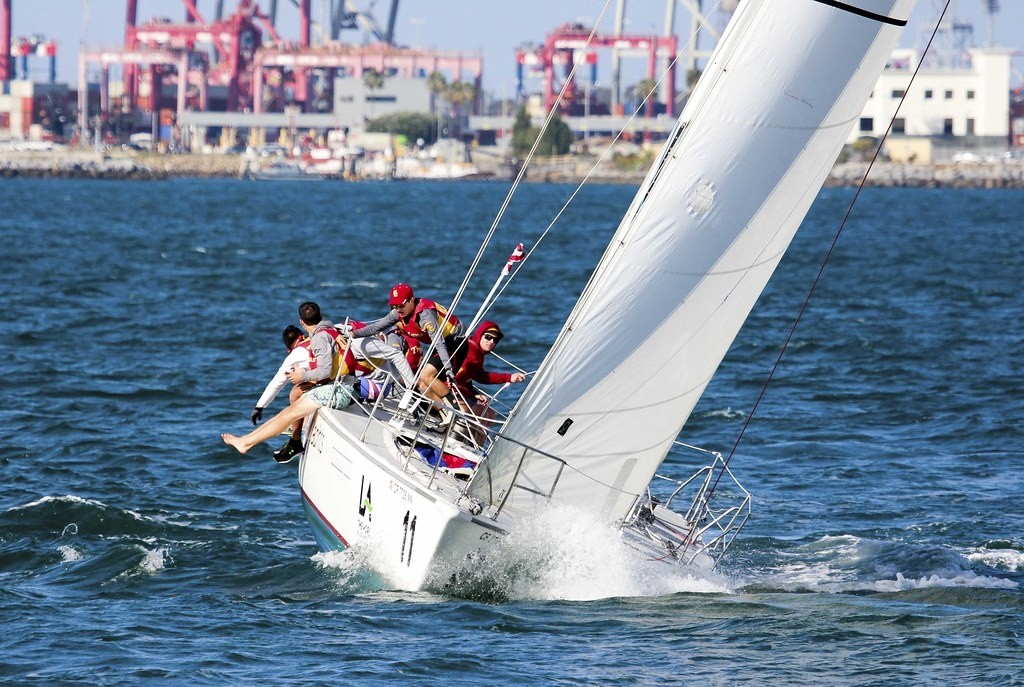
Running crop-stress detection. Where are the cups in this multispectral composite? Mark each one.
[450,418,467,442]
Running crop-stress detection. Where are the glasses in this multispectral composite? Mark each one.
[484,333,499,343]
[392,299,410,309]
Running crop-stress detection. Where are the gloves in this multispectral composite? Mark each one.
[250,408,263,427]
[445,368,457,388]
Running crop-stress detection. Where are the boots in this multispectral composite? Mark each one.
[438,395,461,427]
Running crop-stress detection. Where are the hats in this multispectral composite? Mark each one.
[486,328,503,339]
[388,283,413,305]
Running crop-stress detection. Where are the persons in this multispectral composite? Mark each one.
[222,282,530,464]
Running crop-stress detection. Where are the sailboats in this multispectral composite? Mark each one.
[298,0,945,602]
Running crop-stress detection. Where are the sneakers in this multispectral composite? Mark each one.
[274,442,305,463]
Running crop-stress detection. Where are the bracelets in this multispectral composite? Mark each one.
[446,367,452,371]
